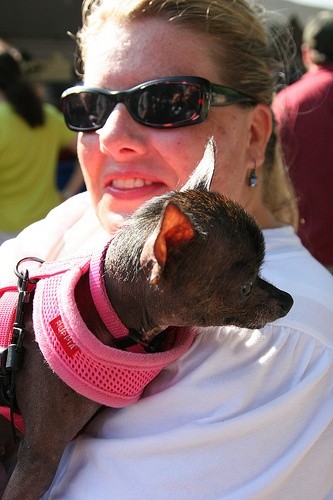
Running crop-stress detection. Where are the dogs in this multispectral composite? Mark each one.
[0,136,294,500]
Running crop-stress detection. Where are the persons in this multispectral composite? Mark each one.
[274,7,332,284]
[0,38,85,247]
[0,0,333,500]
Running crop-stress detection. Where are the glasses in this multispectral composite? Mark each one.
[61,76,262,133]
